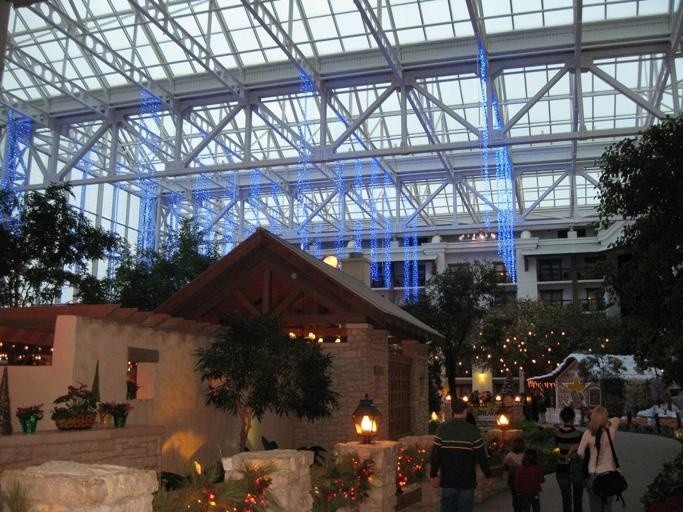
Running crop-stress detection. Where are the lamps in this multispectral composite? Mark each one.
[351,393,383,444]
[495,400,511,426]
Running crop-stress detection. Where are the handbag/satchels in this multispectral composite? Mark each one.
[593,471,627,499]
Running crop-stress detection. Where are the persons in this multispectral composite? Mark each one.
[427,397,494,511]
[513,448,546,511]
[500,438,527,511]
[551,406,588,511]
[576,405,621,511]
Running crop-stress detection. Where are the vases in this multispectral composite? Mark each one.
[19,415,37,433]
[54,413,96,429]
[113,414,127,427]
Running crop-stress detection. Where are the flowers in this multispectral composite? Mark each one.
[15,401,43,418]
[50,383,98,416]
[102,402,133,415]
